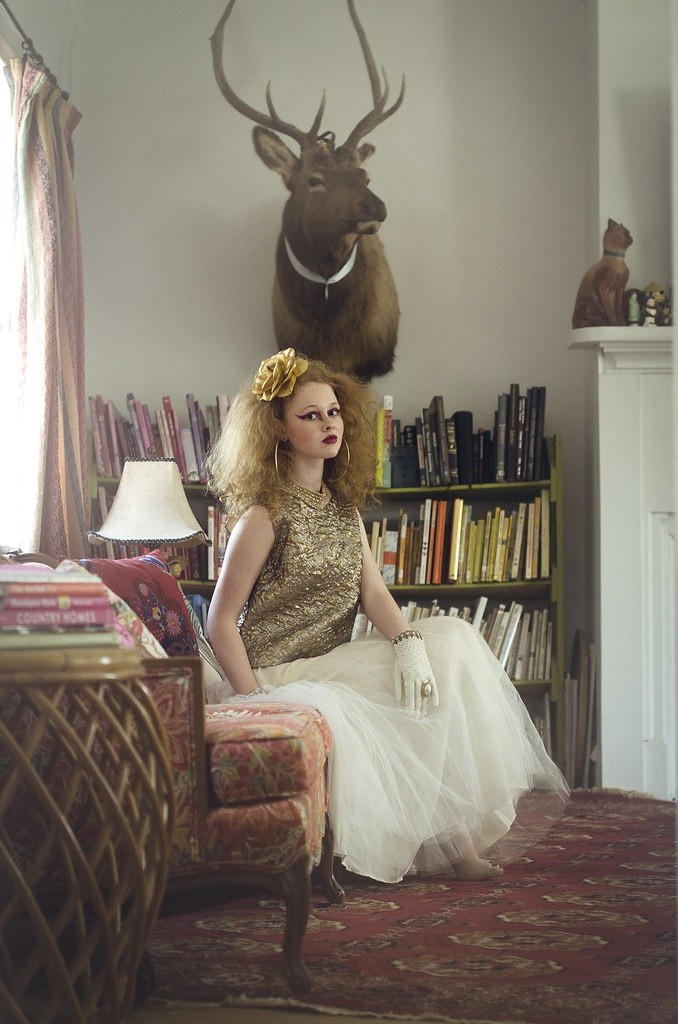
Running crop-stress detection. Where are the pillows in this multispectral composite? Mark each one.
[0,547,199,660]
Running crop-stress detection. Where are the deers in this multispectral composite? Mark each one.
[207,0,407,379]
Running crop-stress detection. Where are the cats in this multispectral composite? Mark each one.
[571,219,634,328]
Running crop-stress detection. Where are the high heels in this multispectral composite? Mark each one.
[416,826,505,882]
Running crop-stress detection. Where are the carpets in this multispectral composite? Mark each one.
[140,786,678,1024]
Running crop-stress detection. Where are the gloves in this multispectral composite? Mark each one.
[392,633,439,719]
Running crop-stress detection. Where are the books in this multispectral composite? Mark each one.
[532,692,552,758]
[88,392,230,485]
[367,383,547,488]
[88,486,233,582]
[350,595,552,682]
[0,572,121,648]
[187,594,210,641]
[364,490,553,587]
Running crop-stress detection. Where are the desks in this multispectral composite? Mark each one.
[0,648,180,1024]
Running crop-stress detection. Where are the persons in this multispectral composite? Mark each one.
[206,348,571,884]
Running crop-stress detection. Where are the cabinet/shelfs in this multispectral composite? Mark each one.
[94,433,569,792]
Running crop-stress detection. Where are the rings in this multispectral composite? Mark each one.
[421,682,433,699]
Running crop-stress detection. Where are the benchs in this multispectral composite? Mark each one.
[14,551,345,992]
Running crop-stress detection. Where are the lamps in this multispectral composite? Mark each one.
[88,456,213,555]
